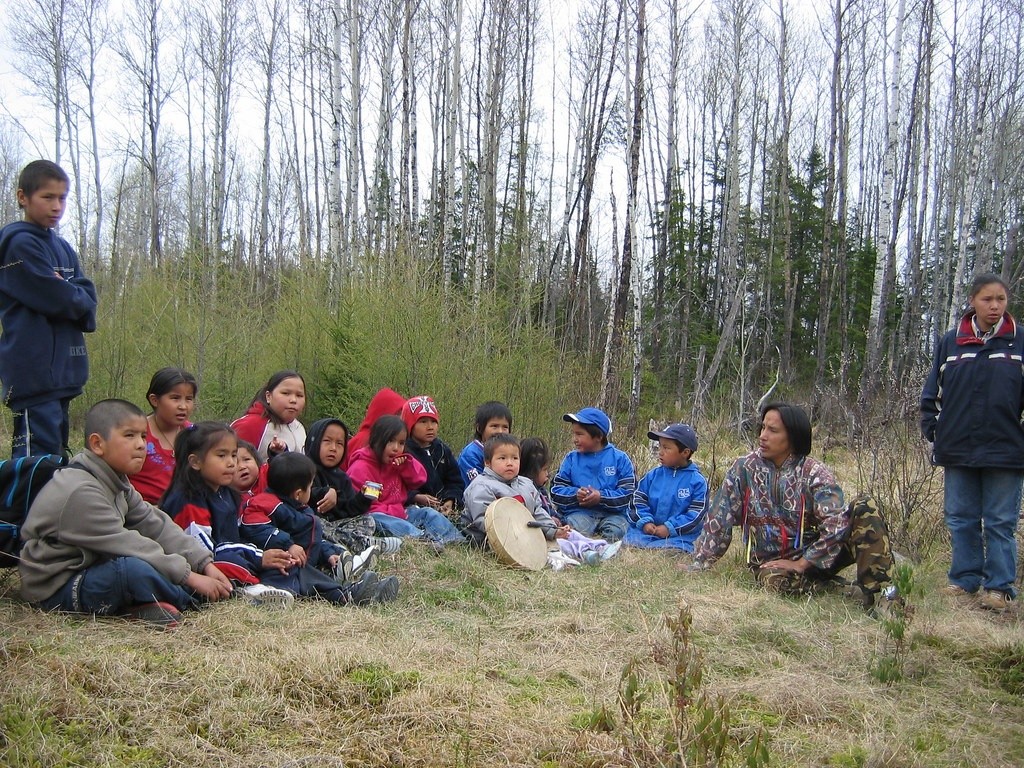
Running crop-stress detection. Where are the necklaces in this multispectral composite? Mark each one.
[153,412,180,458]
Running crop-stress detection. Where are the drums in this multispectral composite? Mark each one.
[484,496,549,571]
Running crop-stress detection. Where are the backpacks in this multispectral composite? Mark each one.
[0,453,96,568]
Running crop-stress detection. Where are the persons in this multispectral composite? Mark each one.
[126,368,623,610]
[0,160,97,460]
[551,408,637,544]
[19,399,233,632]
[626,424,709,554]
[919,273,1024,614]
[678,403,912,627]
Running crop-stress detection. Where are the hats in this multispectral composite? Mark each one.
[563,408,612,437]
[401,396,438,436]
[647,423,698,451]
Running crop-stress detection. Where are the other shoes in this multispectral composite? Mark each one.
[583,549,599,566]
[599,541,623,559]
[338,571,399,607]
[361,535,402,553]
[331,545,381,587]
[119,602,179,625]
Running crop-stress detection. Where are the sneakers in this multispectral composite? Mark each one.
[982,590,1011,611]
[942,584,967,597]
[230,584,294,607]
